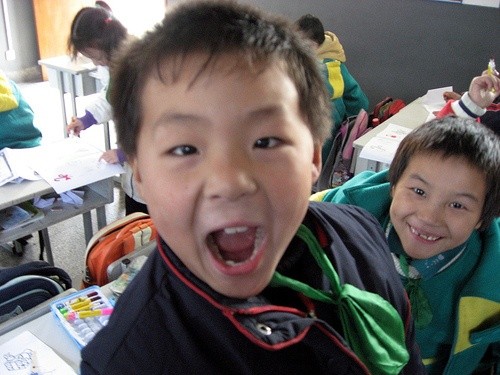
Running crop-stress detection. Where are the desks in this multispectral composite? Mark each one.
[350,97,429,177]
[0,175,114,266]
[37,52,111,152]
[0,283,109,375]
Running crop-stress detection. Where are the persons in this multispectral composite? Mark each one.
[436,69,500,139]
[309,116,500,375]
[80,0,428,375]
[0,70,43,247]
[293,14,369,126]
[66,1,149,217]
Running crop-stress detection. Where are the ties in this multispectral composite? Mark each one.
[272,225,409,375]
[400,255,433,330]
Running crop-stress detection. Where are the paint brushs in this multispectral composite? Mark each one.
[488,54,496,74]
[54,291,114,322]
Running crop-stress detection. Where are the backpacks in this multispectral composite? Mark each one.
[0,260,72,325]
[317,108,373,192]
[373,97,406,124]
[79,212,158,291]
